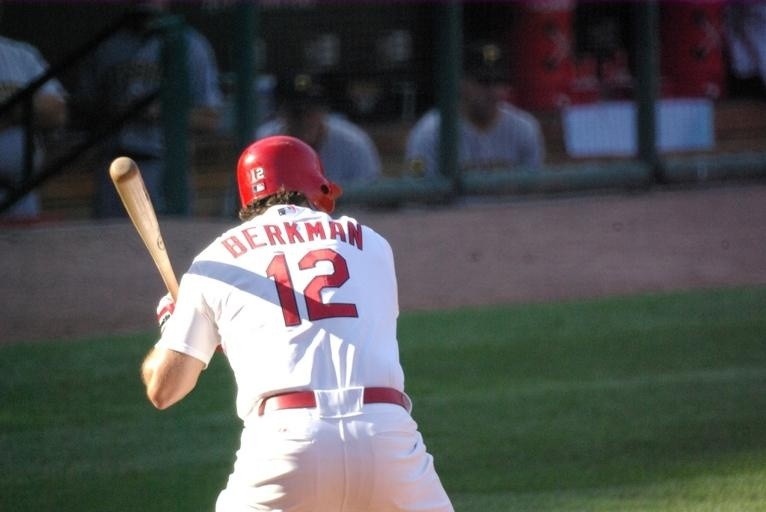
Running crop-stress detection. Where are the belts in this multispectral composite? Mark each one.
[256,386,409,415]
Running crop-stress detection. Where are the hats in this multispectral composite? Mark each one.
[273,65,328,111]
[463,31,518,85]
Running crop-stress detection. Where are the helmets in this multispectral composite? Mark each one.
[235,134,343,215]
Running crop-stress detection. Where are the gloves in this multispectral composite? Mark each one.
[155,290,176,334]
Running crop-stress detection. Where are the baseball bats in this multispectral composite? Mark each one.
[109,158,177,299]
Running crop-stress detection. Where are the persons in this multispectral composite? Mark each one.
[0,37,71,220]
[253,70,381,182]
[78,0,226,216]
[405,42,547,178]
[139,135,455,512]
